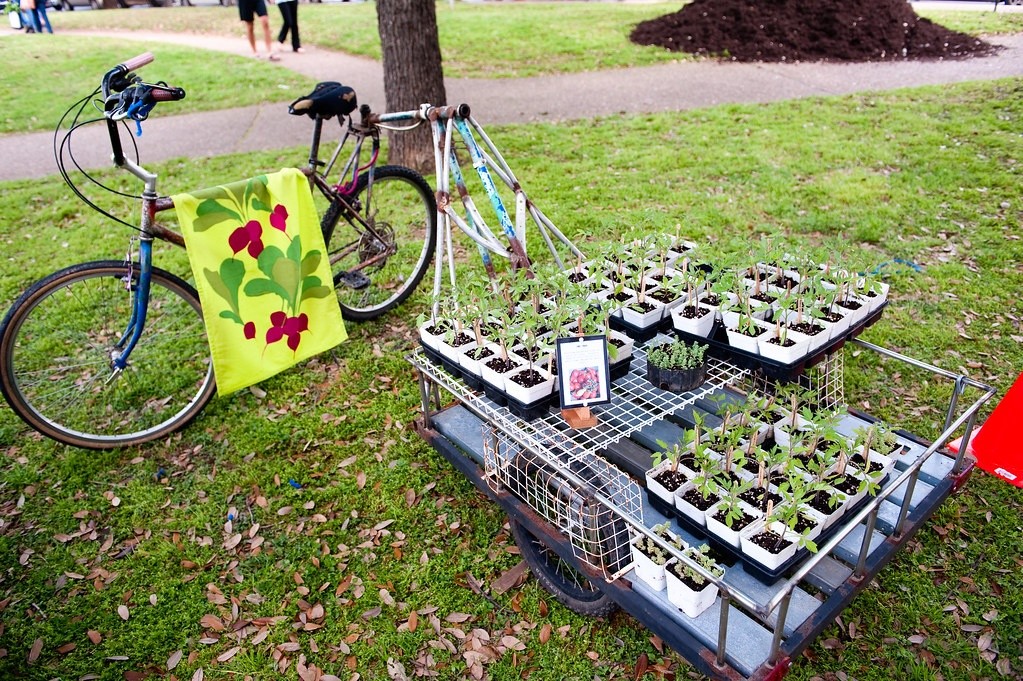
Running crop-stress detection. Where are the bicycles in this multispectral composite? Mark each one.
[0,52,441,452]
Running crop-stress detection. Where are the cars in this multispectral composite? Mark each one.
[0,0,175,13]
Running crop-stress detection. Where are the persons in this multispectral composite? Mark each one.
[239,0,281,61]
[13,0,53,33]
[275,0,305,53]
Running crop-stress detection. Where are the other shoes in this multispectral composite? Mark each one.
[275,40,284,51]
[296,47,304,54]
[254,54,264,60]
[268,54,282,61]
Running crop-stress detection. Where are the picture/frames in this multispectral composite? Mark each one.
[555,335,611,410]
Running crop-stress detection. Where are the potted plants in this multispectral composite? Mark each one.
[420,230,905,619]
